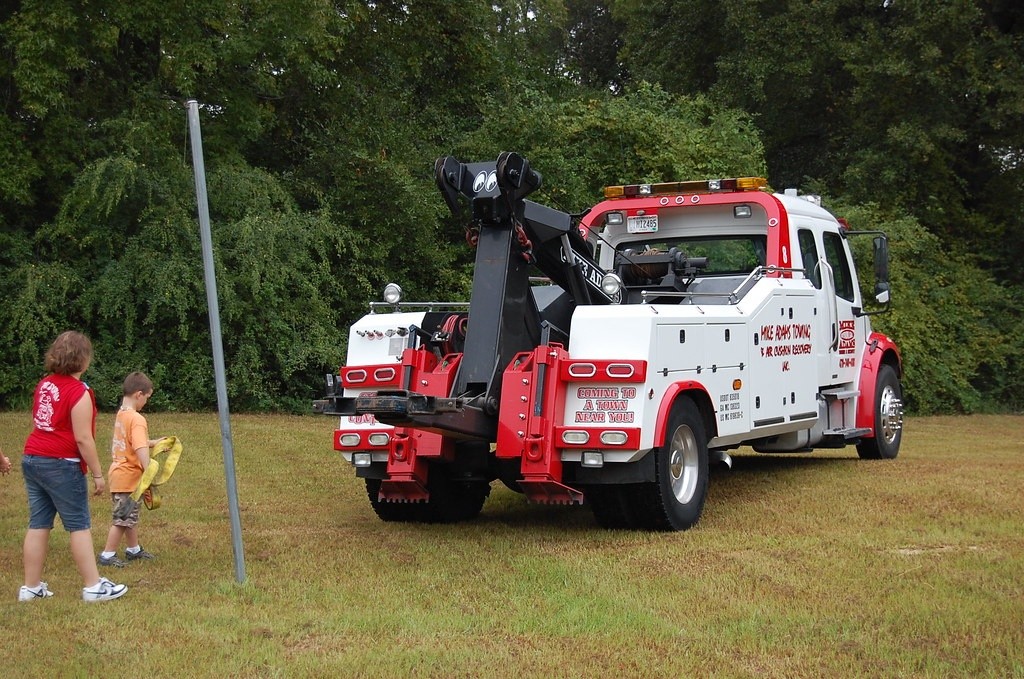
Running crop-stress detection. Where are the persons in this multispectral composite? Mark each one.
[18,331,128,603]
[0,447,12,477]
[98,372,172,569]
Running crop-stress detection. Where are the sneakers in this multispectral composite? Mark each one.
[99,551,129,568]
[125,545,155,561]
[83,577,128,601]
[18,582,54,602]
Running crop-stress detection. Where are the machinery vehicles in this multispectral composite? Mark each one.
[309,153,905,532]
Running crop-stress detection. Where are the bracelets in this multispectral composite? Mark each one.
[91,471,102,478]
